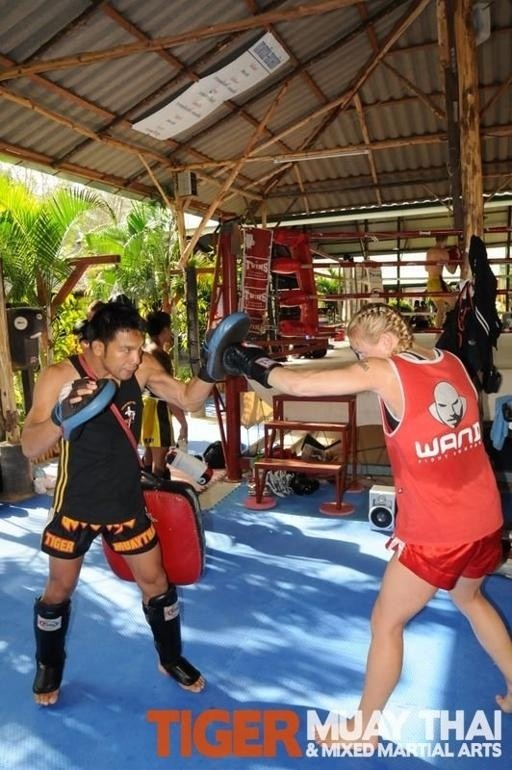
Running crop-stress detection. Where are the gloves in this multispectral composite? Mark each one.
[222,340,282,389]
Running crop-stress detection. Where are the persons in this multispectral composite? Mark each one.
[220,298,512,752]
[426,234,504,440]
[18,291,255,709]
[137,307,189,482]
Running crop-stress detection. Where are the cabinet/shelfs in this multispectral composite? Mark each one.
[245,393,364,515]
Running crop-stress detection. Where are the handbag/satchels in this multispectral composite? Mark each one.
[102,469,205,586]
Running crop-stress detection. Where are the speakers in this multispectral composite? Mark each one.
[368,484,397,532]
[177,172,197,196]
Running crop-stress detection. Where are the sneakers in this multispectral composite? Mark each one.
[265,469,295,498]
